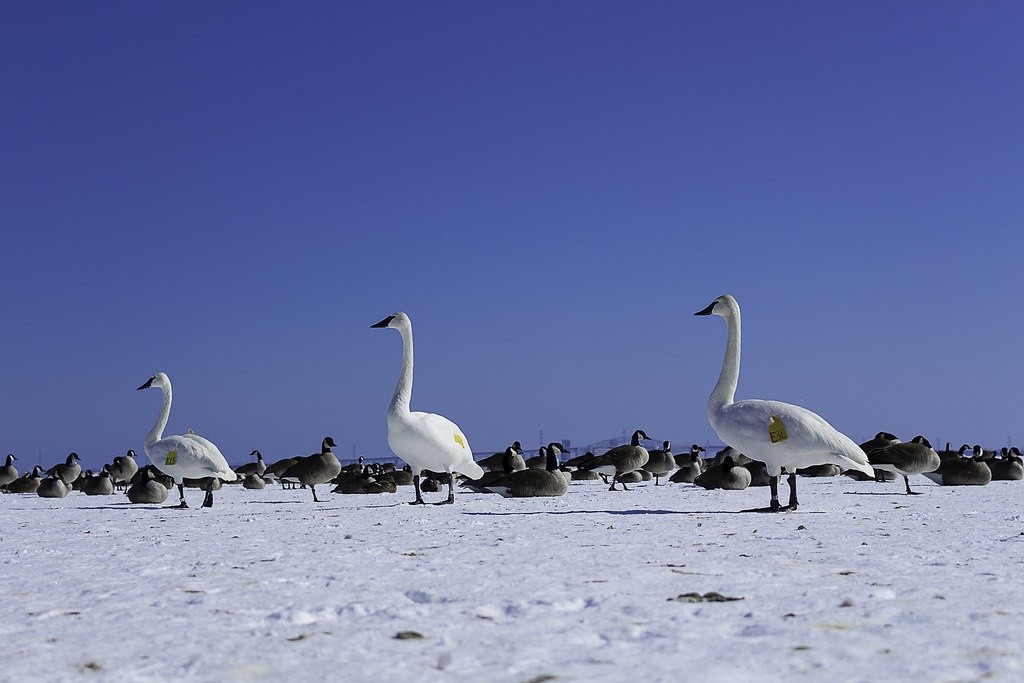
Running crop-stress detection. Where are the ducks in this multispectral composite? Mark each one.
[137,373,237,509]
[369,311,485,506]
[694,294,876,513]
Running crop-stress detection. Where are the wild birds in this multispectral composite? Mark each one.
[922,442,992,486]
[859,432,941,496]
[0,454,18,489]
[643,440,676,486]
[986,446,1024,481]
[8,465,44,494]
[330,456,461,494]
[781,463,898,483]
[481,442,571,497]
[45,452,81,482]
[456,440,601,493]
[237,450,280,490]
[37,467,72,498]
[72,449,223,503]
[279,437,341,502]
[584,429,652,492]
[668,444,781,490]
